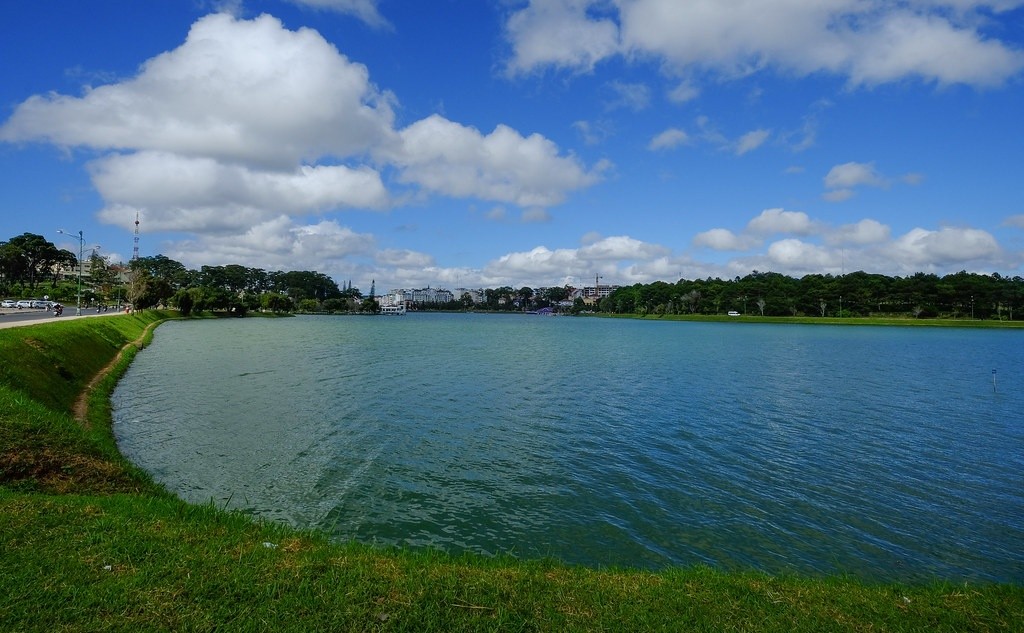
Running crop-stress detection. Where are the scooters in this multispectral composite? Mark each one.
[96,307,107,313]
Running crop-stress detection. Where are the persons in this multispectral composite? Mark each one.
[30,301,107,312]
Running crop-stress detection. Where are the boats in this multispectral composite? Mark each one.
[381,307,404,316]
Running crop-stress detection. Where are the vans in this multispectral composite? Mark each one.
[728,311,740,316]
[17,300,62,317]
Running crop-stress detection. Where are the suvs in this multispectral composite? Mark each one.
[1,300,16,308]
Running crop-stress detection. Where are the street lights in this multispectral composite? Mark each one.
[56,229,101,316]
